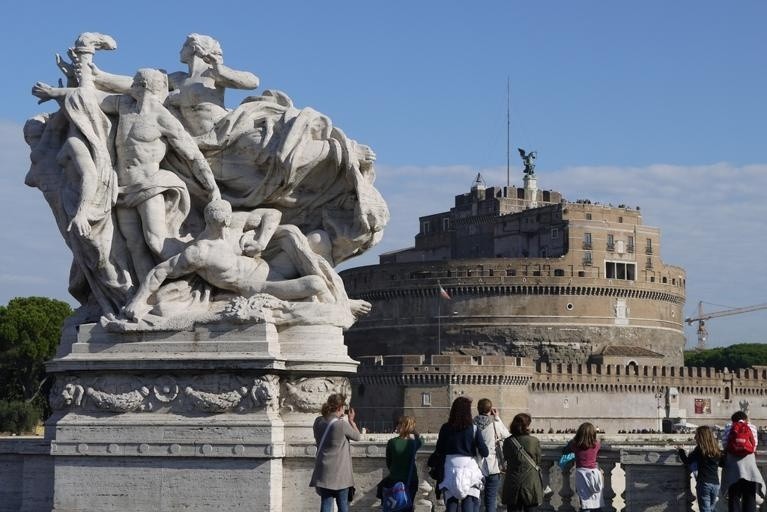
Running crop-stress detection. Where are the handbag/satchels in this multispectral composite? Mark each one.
[309,459,318,487]
[480,457,490,490]
[495,439,508,471]
[381,480,412,512]
[427,448,445,480]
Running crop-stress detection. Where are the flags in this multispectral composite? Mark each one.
[437,279,453,302]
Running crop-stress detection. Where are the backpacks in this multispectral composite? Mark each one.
[729,420,756,455]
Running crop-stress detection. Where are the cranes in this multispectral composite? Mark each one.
[685,301,767,350]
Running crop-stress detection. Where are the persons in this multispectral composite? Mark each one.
[93,33,375,211]
[310,392,360,511]
[125,201,372,322]
[673,422,725,511]
[716,410,766,511]
[26,36,136,317]
[499,412,544,512]
[384,415,424,511]
[33,69,221,283]
[436,394,488,512]
[561,421,603,510]
[471,397,513,511]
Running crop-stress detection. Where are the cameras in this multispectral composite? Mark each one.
[344,410,349,414]
[491,409,496,414]
